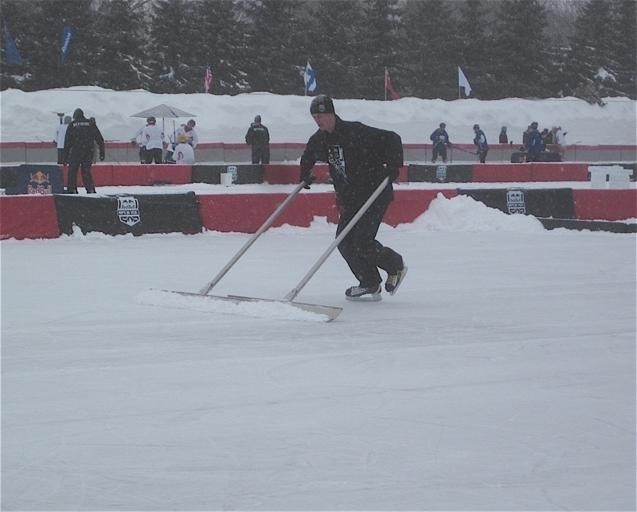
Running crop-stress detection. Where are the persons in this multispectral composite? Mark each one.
[134,117,199,165]
[298,94,403,299]
[499,126,508,144]
[473,124,489,163]
[430,123,451,163]
[245,115,270,164]
[53,108,105,194]
[520,122,567,163]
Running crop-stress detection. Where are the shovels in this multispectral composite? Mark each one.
[148,172,392,322]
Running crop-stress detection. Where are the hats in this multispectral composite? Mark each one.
[309,94,334,114]
[439,122,562,133]
[141,116,196,143]
[73,108,84,120]
[254,115,262,123]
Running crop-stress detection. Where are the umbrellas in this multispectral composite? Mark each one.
[129,104,199,135]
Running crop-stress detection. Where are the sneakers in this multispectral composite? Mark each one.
[345,280,382,296]
[384,264,404,293]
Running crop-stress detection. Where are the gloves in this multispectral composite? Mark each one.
[377,166,400,184]
[300,169,316,190]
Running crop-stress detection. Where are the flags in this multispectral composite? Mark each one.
[303,61,317,92]
[458,66,472,97]
[385,70,400,100]
[204,66,212,90]
